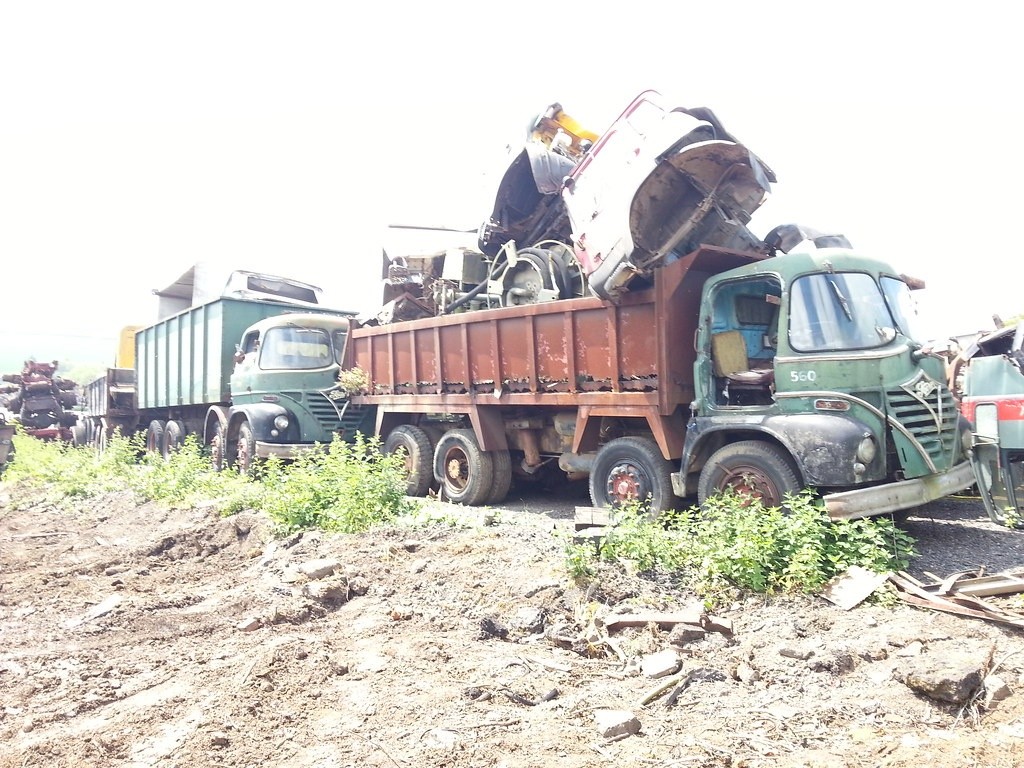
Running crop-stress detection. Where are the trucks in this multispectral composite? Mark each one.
[342,226,983,533]
[133,293,360,468]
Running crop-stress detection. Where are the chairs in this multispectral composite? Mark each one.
[711,330,774,390]
